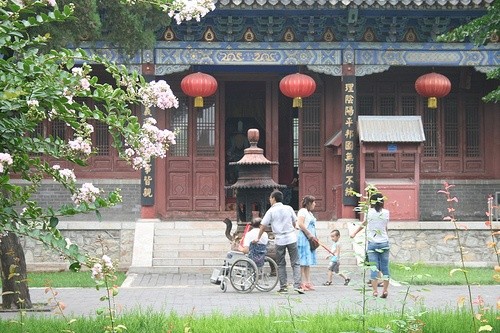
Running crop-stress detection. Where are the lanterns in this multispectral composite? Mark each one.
[415,72,452,108]
[279,73,316,108]
[180,71,218,107]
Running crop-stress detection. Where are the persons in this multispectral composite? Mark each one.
[266,201,272,210]
[322,230,351,286]
[225,200,233,211]
[211,218,268,285]
[255,202,261,218]
[350,188,391,298]
[253,190,306,295]
[296,195,319,291]
[238,200,247,221]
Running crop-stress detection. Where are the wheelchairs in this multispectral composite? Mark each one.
[209,238,280,295]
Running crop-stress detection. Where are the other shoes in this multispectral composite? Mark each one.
[295,288,306,294]
[277,288,288,294]
[305,284,315,290]
[301,284,309,290]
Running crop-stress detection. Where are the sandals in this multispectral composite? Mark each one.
[373,291,378,297]
[380,290,388,298]
[322,281,333,286]
[344,277,351,285]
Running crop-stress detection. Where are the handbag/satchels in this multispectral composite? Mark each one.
[310,238,319,251]
